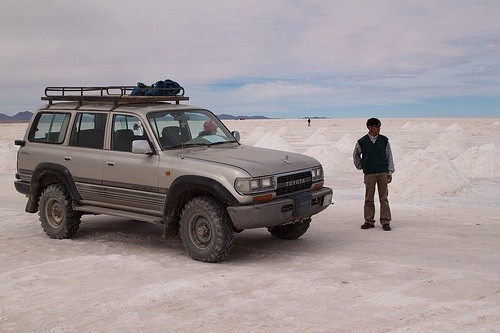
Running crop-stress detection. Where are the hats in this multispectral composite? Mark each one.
[203,121,220,128]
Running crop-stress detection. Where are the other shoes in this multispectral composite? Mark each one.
[361,223,374,229]
[383,224,391,231]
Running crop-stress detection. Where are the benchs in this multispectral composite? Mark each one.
[46,129,103,149]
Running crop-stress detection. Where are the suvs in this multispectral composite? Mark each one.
[13,88,334,263]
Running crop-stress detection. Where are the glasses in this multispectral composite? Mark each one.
[371,124,381,128]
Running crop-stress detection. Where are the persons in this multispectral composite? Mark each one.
[196,120,218,139]
[307,118,310,126]
[353,118,395,231]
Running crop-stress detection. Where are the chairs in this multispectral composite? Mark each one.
[112,130,135,152]
[159,126,186,147]
